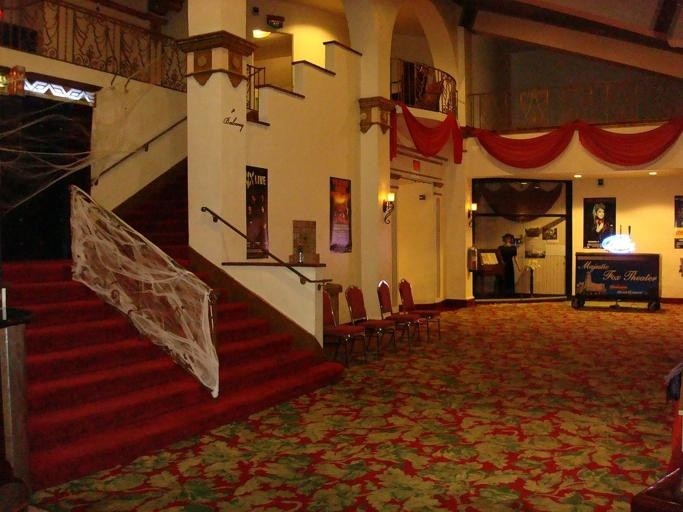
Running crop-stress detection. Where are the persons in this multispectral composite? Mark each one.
[589,201,612,240]
[493,233,520,298]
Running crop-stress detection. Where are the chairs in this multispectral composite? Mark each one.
[322,278,442,368]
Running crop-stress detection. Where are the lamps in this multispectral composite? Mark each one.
[253,29,272,39]
[380,192,397,224]
[468,203,478,227]
